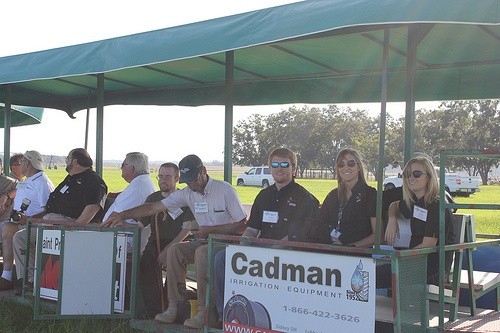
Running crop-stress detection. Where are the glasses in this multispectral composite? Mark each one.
[270,162,292,169]
[402,169,427,179]
[337,160,357,168]
[121,162,128,168]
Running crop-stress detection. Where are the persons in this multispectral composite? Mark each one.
[0,150,55,290]
[12,148,109,298]
[314,149,385,248]
[211,148,320,329]
[101,151,157,256]
[114,163,195,320]
[98,155,247,328]
[375,156,456,297]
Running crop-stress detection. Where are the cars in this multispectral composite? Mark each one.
[236,166,276,188]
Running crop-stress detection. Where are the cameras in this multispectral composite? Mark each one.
[10,198,31,221]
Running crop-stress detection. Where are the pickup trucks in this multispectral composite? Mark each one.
[384,165,483,198]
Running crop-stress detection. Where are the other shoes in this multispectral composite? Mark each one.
[153,303,184,323]
[183,308,206,329]
[0,276,14,291]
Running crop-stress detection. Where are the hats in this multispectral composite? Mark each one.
[178,154,204,183]
[23,150,46,172]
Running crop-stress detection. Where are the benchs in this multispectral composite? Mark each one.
[428,212,500,322]
